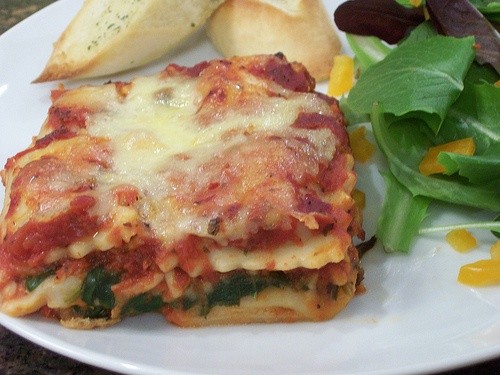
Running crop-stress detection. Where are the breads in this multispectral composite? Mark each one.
[29,1,341,89]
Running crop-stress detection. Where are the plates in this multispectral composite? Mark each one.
[1,0,500,375]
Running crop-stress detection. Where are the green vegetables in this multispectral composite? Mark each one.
[333,0,499,253]
